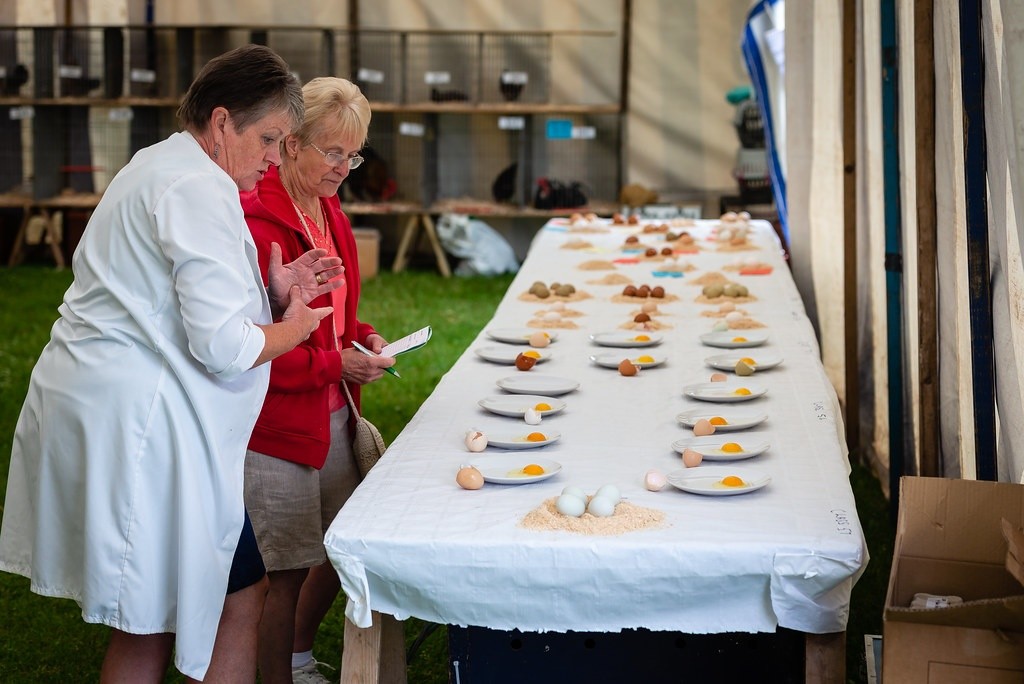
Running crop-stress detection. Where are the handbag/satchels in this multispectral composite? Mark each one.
[352,415,387,478]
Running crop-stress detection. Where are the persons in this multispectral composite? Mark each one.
[239,76,397,684]
[0,44,344,684]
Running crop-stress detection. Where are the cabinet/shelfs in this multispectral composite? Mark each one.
[0,23,623,280]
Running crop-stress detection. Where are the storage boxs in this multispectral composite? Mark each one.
[352,227,380,280]
[879,474,1023,683]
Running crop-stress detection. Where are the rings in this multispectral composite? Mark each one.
[315,273,328,285]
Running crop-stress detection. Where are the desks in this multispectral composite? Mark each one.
[321,215,871,683]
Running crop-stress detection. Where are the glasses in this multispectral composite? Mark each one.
[310,139,364,170]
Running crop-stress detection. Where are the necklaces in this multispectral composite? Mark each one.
[279,171,321,229]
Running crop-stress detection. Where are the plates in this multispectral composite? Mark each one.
[666,466,770,495]
[484,430,561,448]
[683,380,767,401]
[589,332,664,346]
[707,350,783,371]
[475,453,562,484]
[486,326,557,345]
[479,396,564,417]
[476,344,551,366]
[676,406,768,430]
[497,375,579,395]
[701,330,769,347]
[589,350,667,371]
[672,434,770,461]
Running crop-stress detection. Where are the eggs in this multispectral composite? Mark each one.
[740,357,756,365]
[736,388,751,395]
[710,417,726,424]
[524,351,542,358]
[714,443,748,454]
[635,335,649,341]
[556,484,623,517]
[513,432,551,442]
[635,356,655,362]
[507,464,546,477]
[536,403,551,410]
[712,476,750,487]
[732,337,747,342]
[530,212,749,330]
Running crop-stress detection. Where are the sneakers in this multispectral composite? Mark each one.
[291,657,335,684]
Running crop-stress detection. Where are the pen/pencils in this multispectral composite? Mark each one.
[351,341,402,378]
